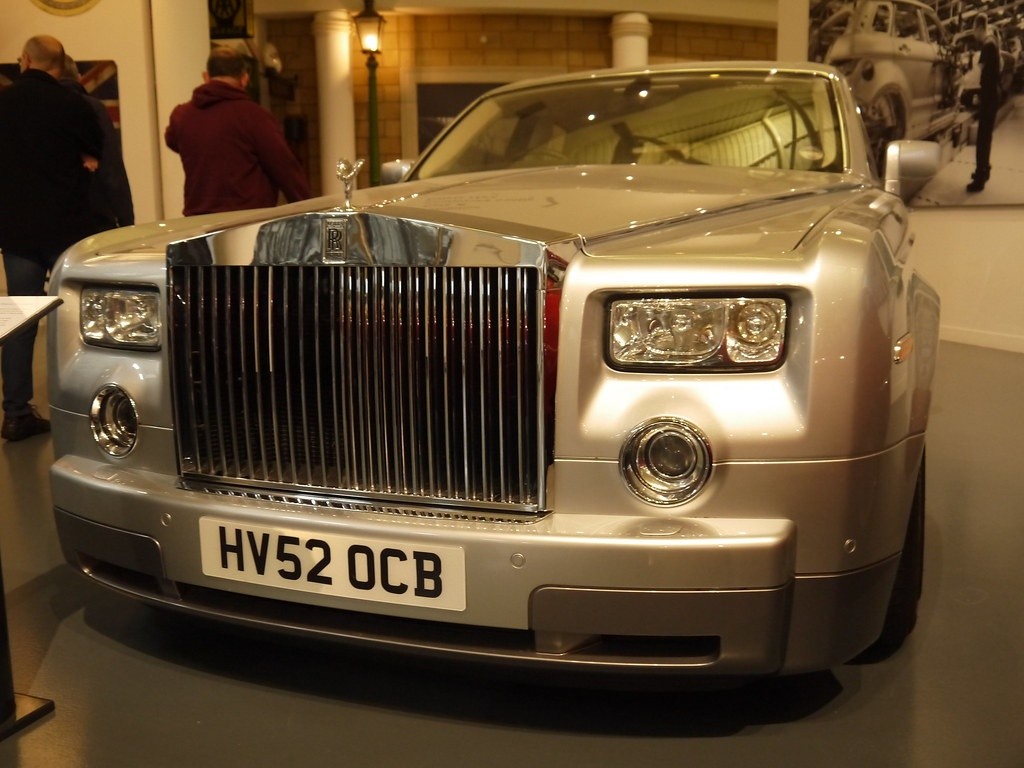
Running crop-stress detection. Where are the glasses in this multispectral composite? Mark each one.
[17,57,23,65]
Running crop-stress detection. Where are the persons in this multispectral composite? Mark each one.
[0,35,134,445]
[965,11,1001,190]
[163,44,310,218]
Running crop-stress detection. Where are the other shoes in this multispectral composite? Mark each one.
[1,406,50,440]
[967,169,985,192]
[971,165,991,182]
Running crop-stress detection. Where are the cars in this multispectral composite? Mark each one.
[48,60,942,676]
[809,1,959,150]
